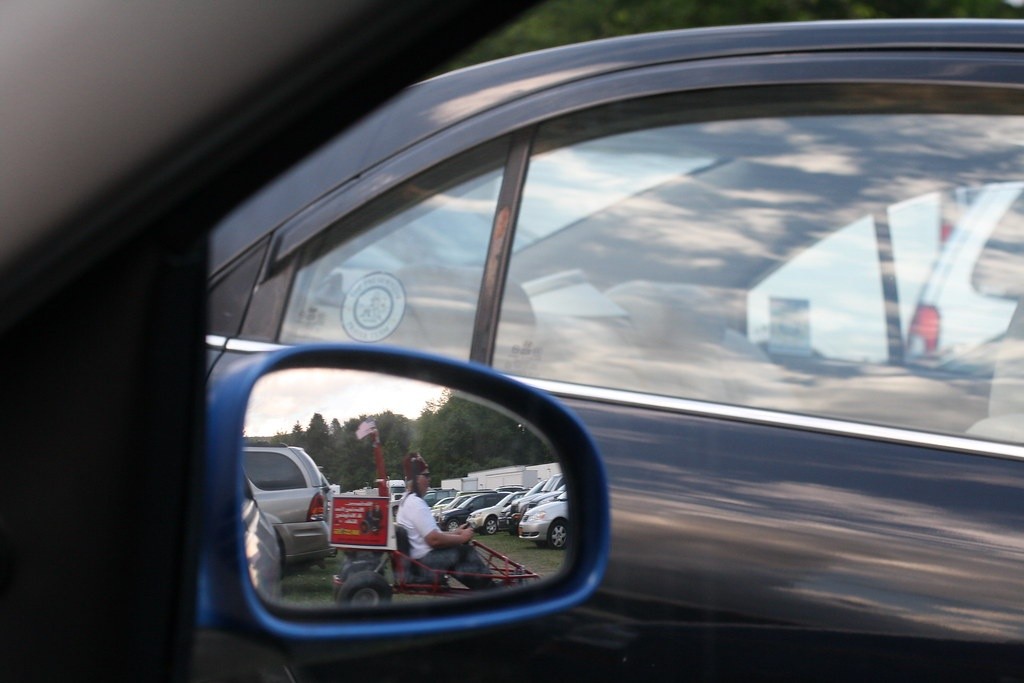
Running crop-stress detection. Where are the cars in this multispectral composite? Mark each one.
[239,441,329,570]
[387,473,570,549]
[195,19,1024,683]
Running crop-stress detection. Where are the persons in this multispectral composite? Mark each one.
[396,451,497,590]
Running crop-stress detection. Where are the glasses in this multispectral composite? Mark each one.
[418,472,430,477]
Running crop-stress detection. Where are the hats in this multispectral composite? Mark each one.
[403,452,428,479]
